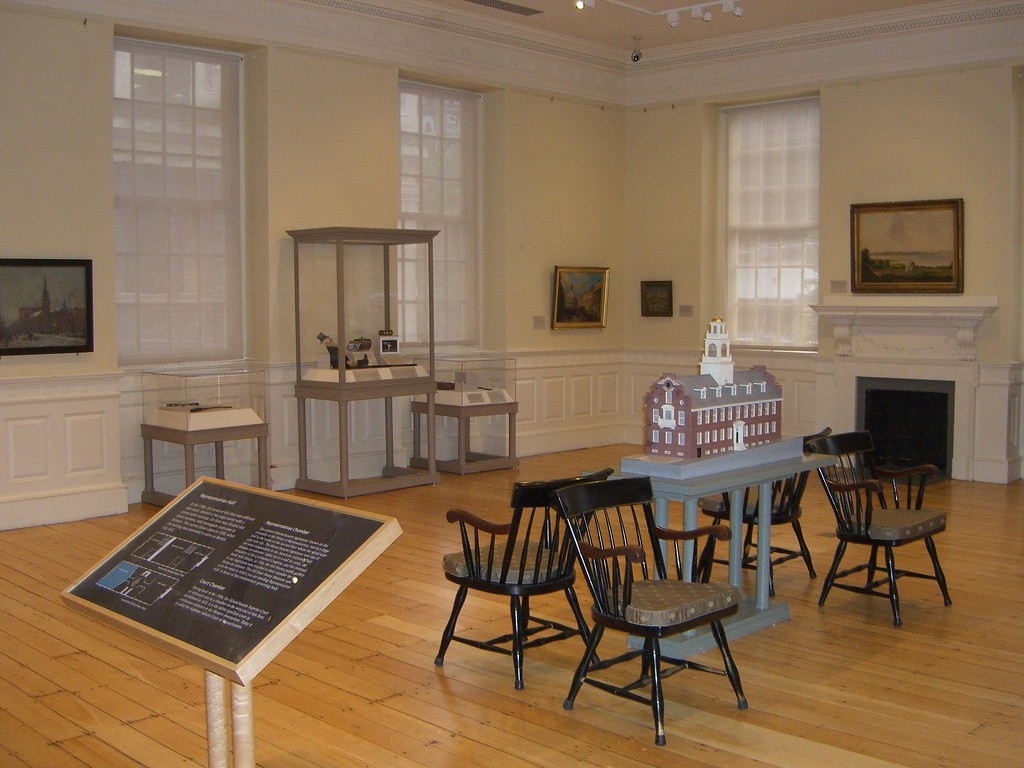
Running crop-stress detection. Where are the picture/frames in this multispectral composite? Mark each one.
[551,266,610,328]
[641,280,673,317]
[850,197,964,294]
[0,258,95,356]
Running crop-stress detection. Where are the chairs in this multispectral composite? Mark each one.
[556,476,748,745]
[806,430,952,626]
[435,467,615,691]
[699,427,833,594]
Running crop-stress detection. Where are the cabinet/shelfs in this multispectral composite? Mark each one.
[286,225,442,499]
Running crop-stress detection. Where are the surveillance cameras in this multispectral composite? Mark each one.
[631,49,641,62]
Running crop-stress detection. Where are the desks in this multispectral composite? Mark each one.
[581,452,840,660]
